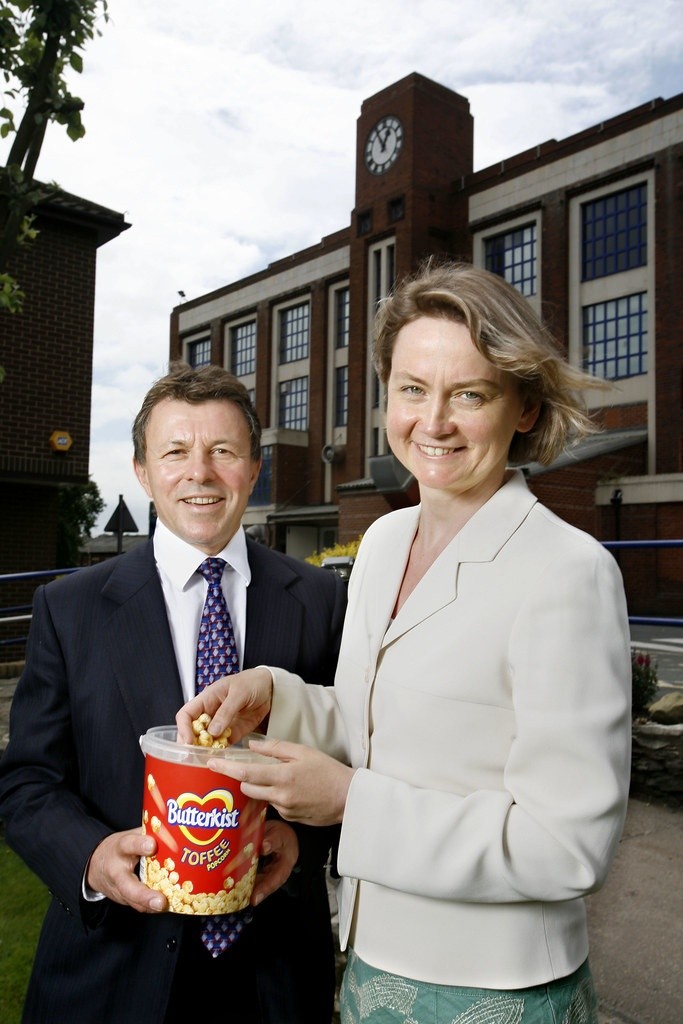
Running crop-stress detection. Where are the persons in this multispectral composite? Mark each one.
[174,261,633,1024]
[0,365,356,1024]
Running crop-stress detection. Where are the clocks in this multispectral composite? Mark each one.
[363,114,406,177]
[332,424,347,446]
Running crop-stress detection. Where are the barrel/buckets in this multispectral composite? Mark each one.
[139,725,280,915]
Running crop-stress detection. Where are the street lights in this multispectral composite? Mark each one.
[611,489,623,563]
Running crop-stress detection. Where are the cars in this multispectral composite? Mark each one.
[320,556,355,584]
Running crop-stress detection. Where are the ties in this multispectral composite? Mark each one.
[196,559,253,957]
